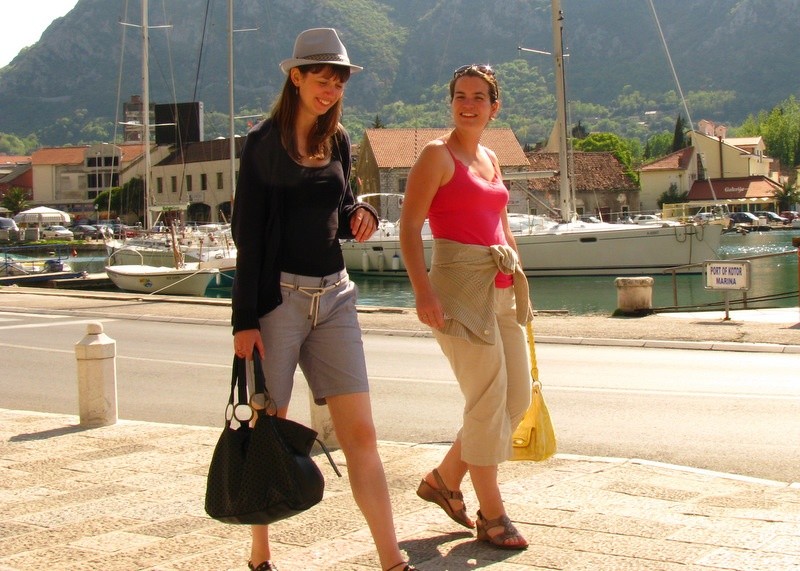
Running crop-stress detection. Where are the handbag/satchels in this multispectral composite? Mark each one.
[506,384,557,461]
[205,345,342,525]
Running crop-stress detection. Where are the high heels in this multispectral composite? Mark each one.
[416,469,475,529]
[476,509,528,549]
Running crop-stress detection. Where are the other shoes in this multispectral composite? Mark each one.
[247,560,278,571]
[385,562,421,571]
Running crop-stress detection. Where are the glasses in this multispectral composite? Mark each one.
[454,64,498,99]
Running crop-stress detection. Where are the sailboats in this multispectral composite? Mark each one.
[99,0,754,279]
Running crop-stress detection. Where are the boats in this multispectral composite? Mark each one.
[104,217,221,298]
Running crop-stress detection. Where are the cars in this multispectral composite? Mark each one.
[111,223,144,240]
[695,212,721,223]
[728,210,800,227]
[151,226,172,234]
[617,214,680,228]
[91,224,114,239]
[41,225,74,241]
[0,217,20,245]
[68,224,98,240]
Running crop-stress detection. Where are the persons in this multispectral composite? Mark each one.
[233,28,415,571]
[398,63,533,552]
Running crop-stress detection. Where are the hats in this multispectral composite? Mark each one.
[279,28,363,75]
